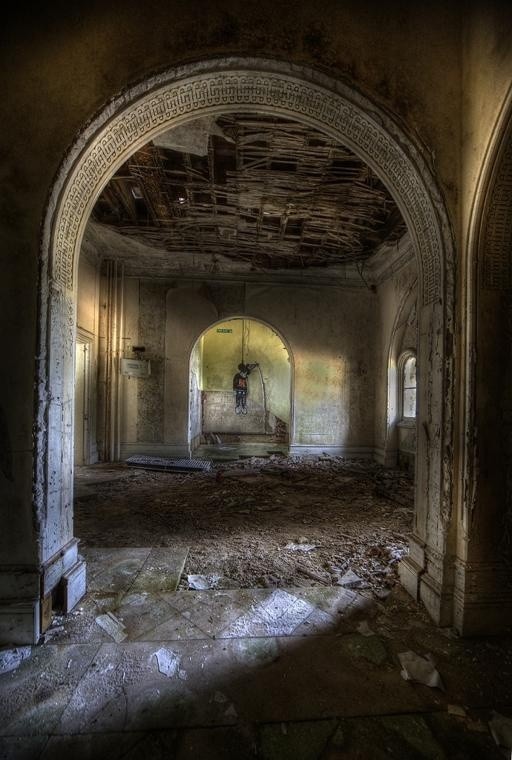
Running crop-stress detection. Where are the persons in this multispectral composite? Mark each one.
[233,364,250,415]
